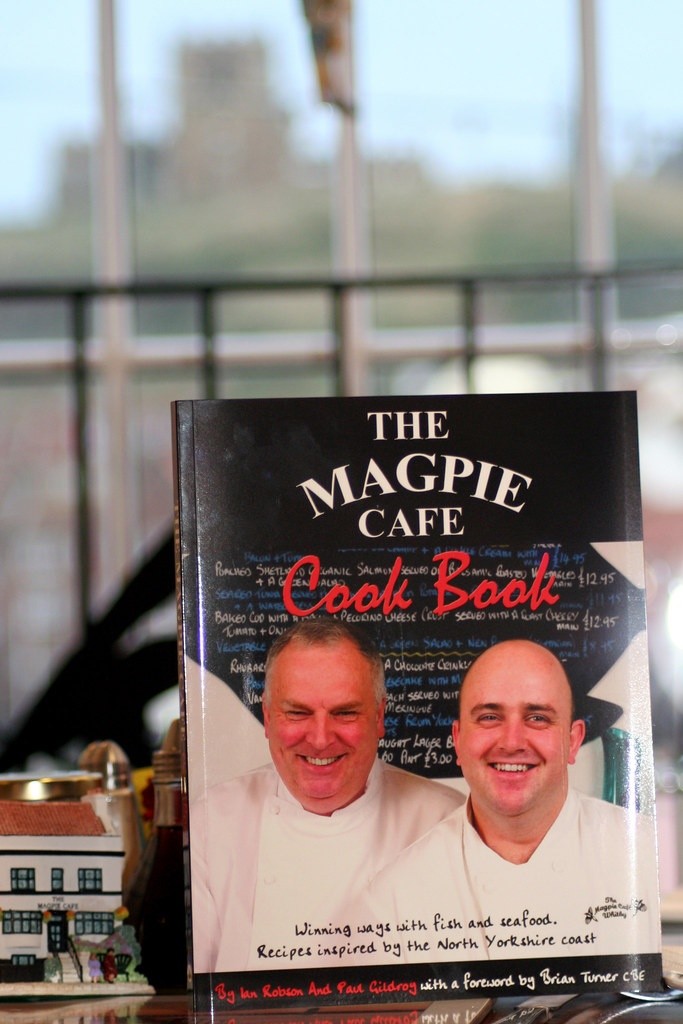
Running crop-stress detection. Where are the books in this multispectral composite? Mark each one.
[171,390,664,1009]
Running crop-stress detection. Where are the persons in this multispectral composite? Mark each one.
[188,618,465,968]
[363,640,660,954]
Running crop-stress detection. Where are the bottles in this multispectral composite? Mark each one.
[122,752,186,992]
